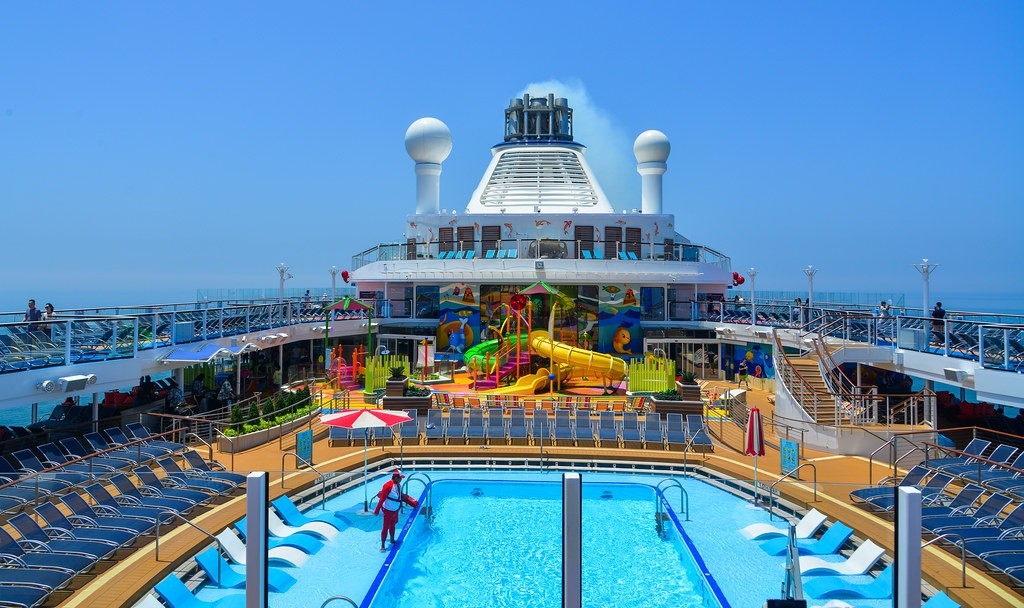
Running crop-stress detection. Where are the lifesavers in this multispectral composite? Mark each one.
[377,491,418,508]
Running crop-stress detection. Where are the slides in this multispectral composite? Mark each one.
[463,330,528,373]
[479,336,628,396]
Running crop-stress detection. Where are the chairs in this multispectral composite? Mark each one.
[268,508,339,542]
[841,590,962,608]
[435,248,519,259]
[155,575,246,607]
[271,495,353,532]
[580,248,640,259]
[133,594,166,608]
[847,438,1024,594]
[233,514,325,556]
[758,521,855,556]
[1,304,370,372]
[0,419,250,608]
[327,390,717,456]
[215,527,309,568]
[782,539,886,574]
[194,547,298,592]
[706,300,1024,374]
[739,507,829,540]
[802,563,895,599]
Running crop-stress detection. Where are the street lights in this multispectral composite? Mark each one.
[276,262,289,321]
[802,265,820,328]
[327,265,339,320]
[746,268,759,326]
[913,259,940,351]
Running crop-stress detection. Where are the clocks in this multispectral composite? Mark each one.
[534,261,545,270]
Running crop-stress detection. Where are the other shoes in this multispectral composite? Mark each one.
[380,541,397,553]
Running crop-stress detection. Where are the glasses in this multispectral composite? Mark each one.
[45,306,48,308]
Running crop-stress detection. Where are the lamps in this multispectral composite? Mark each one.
[712,326,734,336]
[36,373,99,396]
[362,322,380,328]
[753,331,772,340]
[262,331,289,346]
[943,368,972,383]
[313,326,334,335]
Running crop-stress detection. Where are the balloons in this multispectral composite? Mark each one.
[733,271,745,286]
[341,271,349,283]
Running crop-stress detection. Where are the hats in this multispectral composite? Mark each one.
[393,469,405,478]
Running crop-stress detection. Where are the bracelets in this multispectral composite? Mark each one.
[38,326,41,327]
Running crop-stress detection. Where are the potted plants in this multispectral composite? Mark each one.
[675,371,702,400]
[385,366,409,396]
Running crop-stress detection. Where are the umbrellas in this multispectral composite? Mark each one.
[318,406,414,512]
[745,405,765,506]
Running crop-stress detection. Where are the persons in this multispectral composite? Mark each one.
[192,373,208,413]
[18,299,57,360]
[738,359,752,390]
[373,469,405,552]
[879,301,895,342]
[371,290,384,316]
[878,373,911,388]
[793,297,809,328]
[322,293,328,307]
[694,292,745,319]
[304,290,310,308]
[165,383,186,414]
[932,302,946,348]
[216,374,236,412]
[704,348,715,375]
[272,365,281,393]
[139,376,153,399]
[62,397,75,406]
[300,353,312,371]
